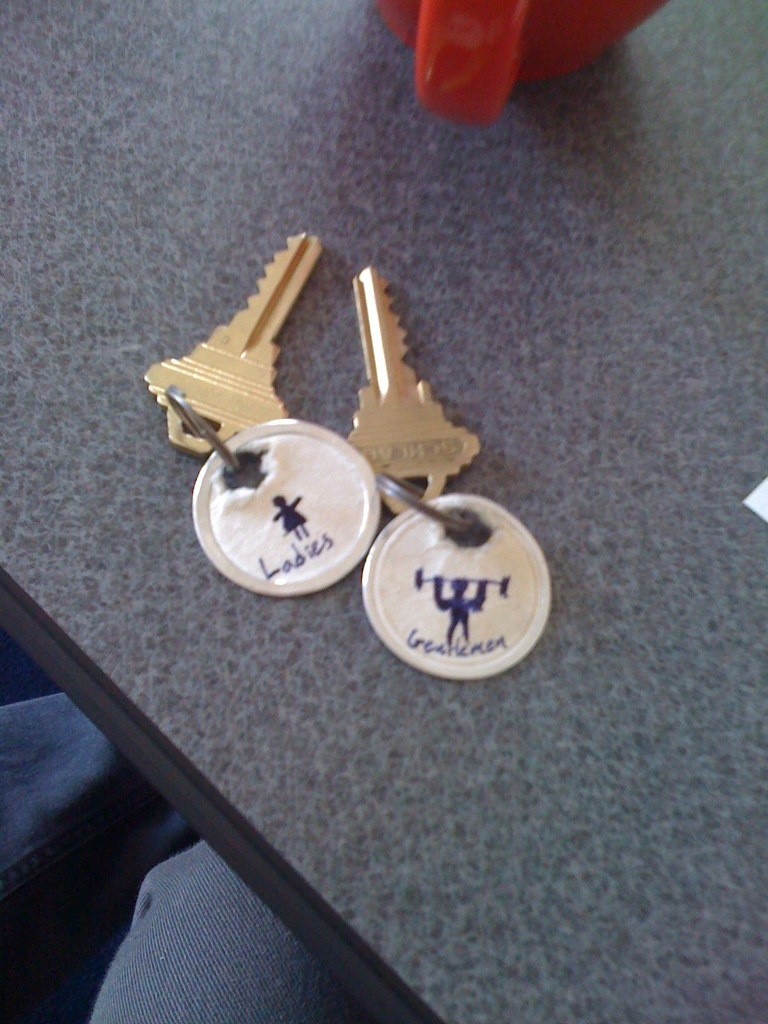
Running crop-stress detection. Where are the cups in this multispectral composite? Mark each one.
[375,0,671,128]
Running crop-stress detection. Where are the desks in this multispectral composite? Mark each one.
[0,1,767,1024]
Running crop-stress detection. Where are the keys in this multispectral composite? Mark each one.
[350,263,477,515]
[145,230,317,460]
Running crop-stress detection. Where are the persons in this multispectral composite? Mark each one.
[0,685,361,1024]
